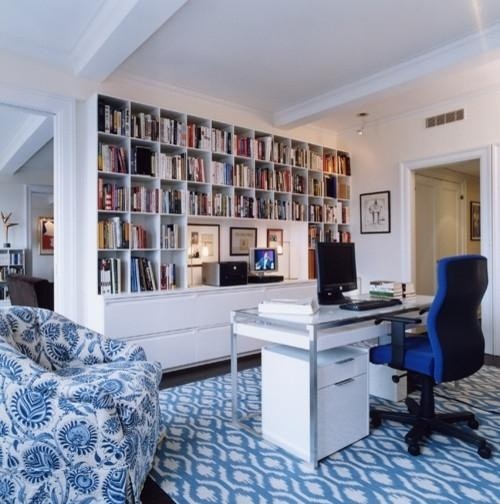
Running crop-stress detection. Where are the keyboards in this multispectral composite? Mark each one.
[340,300,392,311]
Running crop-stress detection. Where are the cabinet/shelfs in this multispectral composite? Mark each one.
[260,342,370,463]
[0,248,26,307]
[80,89,352,299]
[84,286,318,374]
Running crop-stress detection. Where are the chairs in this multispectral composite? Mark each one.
[0,298,167,504]
[364,251,496,460]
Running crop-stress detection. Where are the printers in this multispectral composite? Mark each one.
[202,261,248,287]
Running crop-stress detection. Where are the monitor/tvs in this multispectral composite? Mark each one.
[248,247,283,283]
[315,242,357,304]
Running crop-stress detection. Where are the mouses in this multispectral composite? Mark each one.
[391,299,402,304]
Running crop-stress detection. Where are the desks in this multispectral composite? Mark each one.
[224,290,442,474]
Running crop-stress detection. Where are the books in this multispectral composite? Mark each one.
[188,122,351,224]
[258,298,320,315]
[309,224,350,249]
[368,280,416,300]
[0,253,24,301]
[98,105,187,294]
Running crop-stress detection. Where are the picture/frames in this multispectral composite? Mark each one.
[266,227,285,255]
[359,190,392,234]
[37,215,54,256]
[229,226,257,256]
[187,223,220,267]
[470,200,481,241]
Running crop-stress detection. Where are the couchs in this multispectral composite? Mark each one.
[6,273,54,311]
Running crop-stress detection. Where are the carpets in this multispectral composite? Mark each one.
[143,359,499,503]
